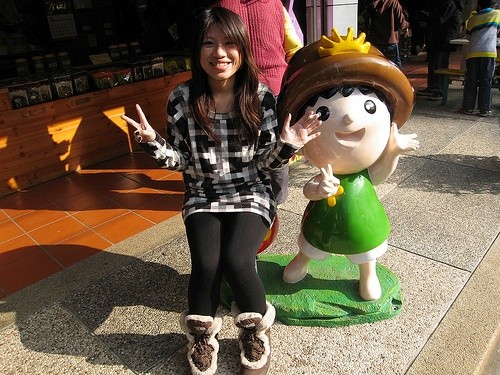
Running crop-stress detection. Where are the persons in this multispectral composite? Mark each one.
[416,0,454,100]
[277,28,419,300]
[460,0,500,116]
[366,0,409,70]
[219,0,301,165]
[121,8,321,375]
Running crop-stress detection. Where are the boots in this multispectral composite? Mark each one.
[231,301,276,374]
[179,308,223,375]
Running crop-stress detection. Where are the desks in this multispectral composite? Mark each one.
[0,51,193,199]
[450,38,500,71]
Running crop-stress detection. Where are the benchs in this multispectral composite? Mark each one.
[433,67,500,105]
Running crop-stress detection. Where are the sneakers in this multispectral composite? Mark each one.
[479,109,494,116]
[417,88,435,96]
[459,107,474,114]
[428,92,443,100]
